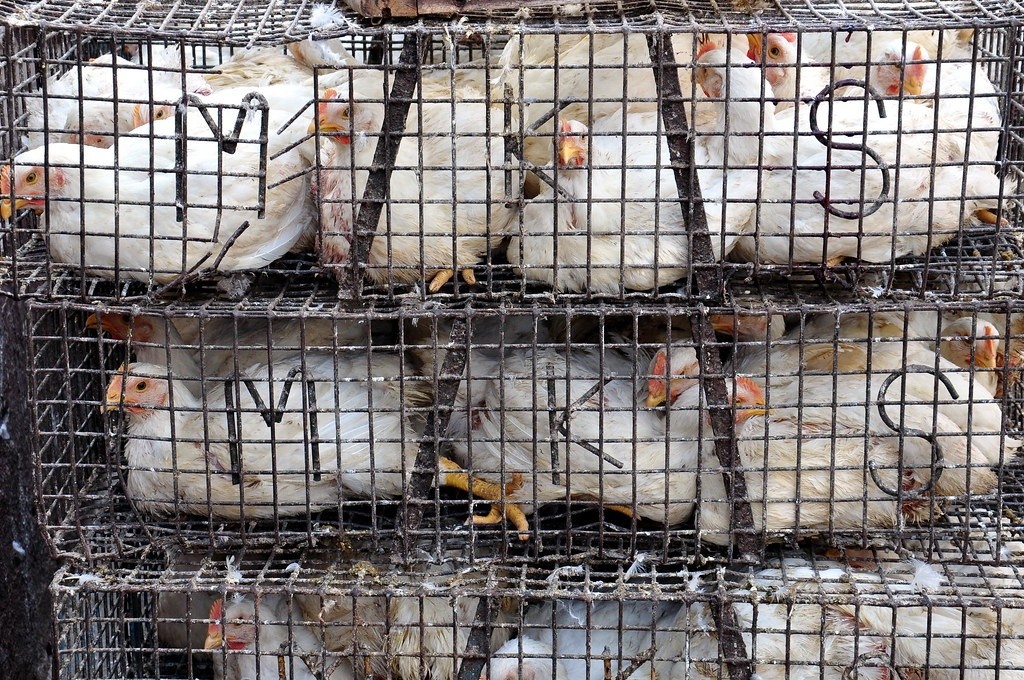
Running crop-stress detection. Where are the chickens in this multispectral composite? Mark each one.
[0,0,1024,680]
